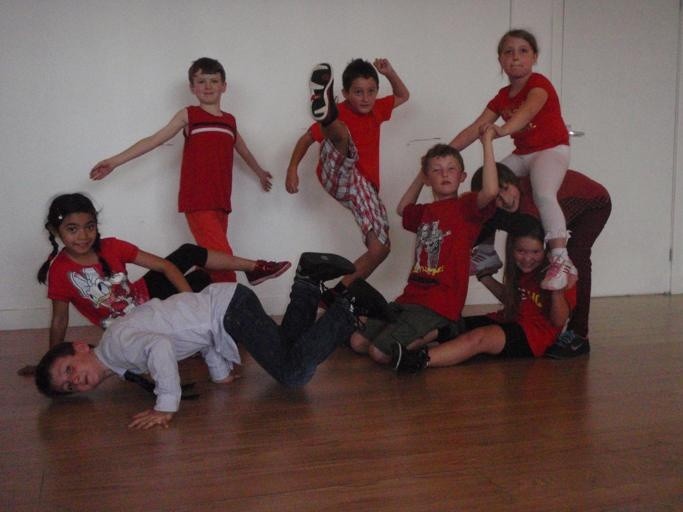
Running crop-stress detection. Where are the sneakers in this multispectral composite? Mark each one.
[310,62,335,125]
[246,260,290,285]
[393,341,427,373]
[346,278,388,318]
[546,331,590,360]
[539,261,578,292]
[469,249,502,272]
[297,252,356,282]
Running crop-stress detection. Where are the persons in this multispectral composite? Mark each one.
[348,128,498,367]
[285,56,409,311]
[391,219,578,368]
[90,58,273,287]
[420,29,580,290]
[13,193,290,379]
[35,251,387,432]
[471,163,612,359]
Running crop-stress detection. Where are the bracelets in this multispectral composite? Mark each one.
[477,273,491,281]
[254,166,260,172]
